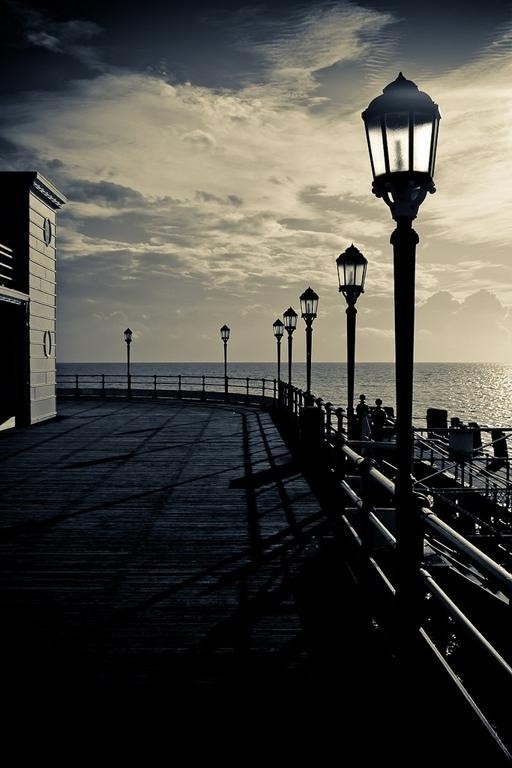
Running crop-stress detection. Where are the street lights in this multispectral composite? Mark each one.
[361,73,442,675]
[124,328,133,399]
[299,286,320,406]
[335,243,368,465]
[272,306,298,414]
[220,324,230,393]
